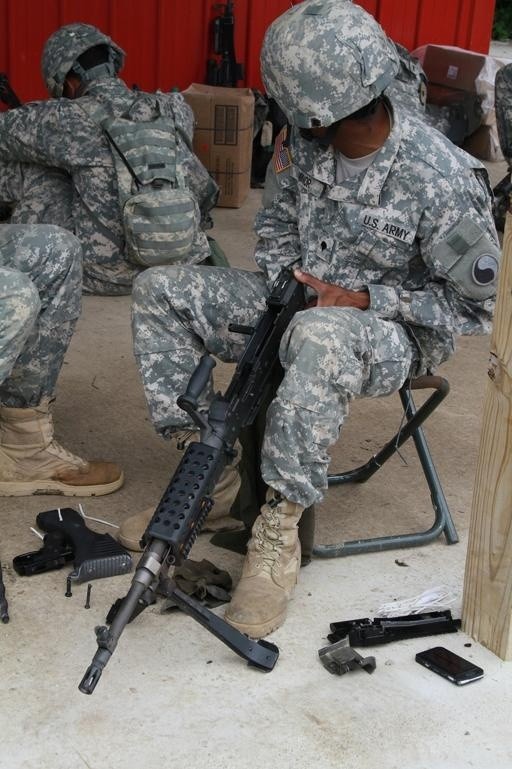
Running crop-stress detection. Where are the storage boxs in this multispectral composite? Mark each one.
[181,82,255,210]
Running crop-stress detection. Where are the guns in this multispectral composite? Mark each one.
[492,173,512,234]
[206,0,244,88]
[78,265,310,694]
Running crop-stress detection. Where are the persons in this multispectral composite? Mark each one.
[0,219,123,499]
[117,0,504,639]
[0,23,221,297]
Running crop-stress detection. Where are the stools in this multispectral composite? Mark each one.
[297,377,460,558]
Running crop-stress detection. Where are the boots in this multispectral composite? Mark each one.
[221,489,306,641]
[0,403,124,498]
[117,443,244,550]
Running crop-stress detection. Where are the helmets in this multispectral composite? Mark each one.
[38,19,128,99]
[256,2,403,132]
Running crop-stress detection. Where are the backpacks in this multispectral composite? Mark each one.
[98,113,203,267]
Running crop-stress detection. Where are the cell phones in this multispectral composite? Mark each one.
[414,646,484,686]
